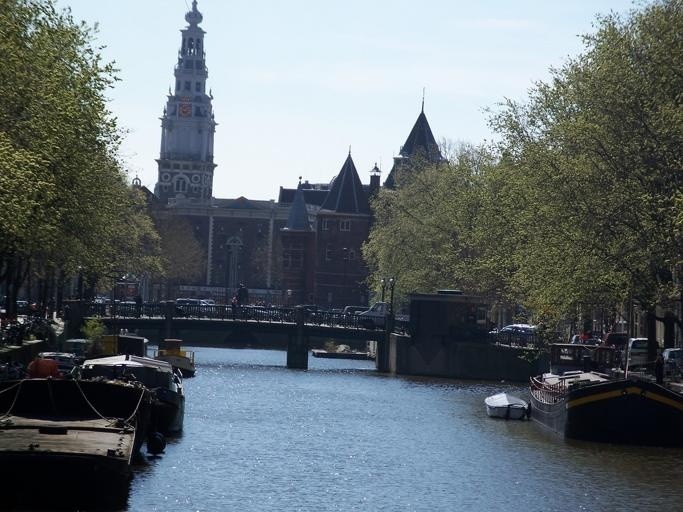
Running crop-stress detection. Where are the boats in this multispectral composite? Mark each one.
[481,391,532,420]
[0,330,195,492]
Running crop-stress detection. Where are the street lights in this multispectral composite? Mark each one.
[381,278,385,302]
[387,276,396,334]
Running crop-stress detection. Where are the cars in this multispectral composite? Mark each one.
[489,324,682,381]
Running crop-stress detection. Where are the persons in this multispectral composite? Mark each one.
[135,292,142,317]
[655,348,664,384]
[232,297,238,319]
[48,296,55,318]
